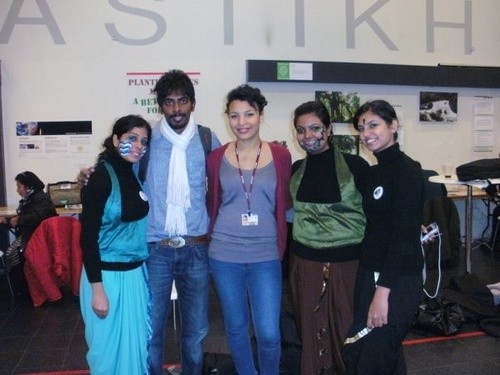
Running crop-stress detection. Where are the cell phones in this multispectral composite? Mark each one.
[420,223,437,242]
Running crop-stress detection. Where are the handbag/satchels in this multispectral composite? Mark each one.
[48,181,82,206]
[455,158,500,181]
[411,295,464,337]
[445,271,496,329]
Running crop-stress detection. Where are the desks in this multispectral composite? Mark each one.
[0,207,82,216]
[428,175,500,272]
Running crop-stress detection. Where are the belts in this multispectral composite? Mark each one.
[152,236,209,246]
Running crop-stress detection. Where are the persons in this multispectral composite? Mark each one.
[205,84,293,375]
[288,101,372,375]
[79,69,288,375]
[349,100,424,375]
[5,171,57,300]
[78,114,155,375]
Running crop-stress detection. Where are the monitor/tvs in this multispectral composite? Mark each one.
[47,182,82,208]
